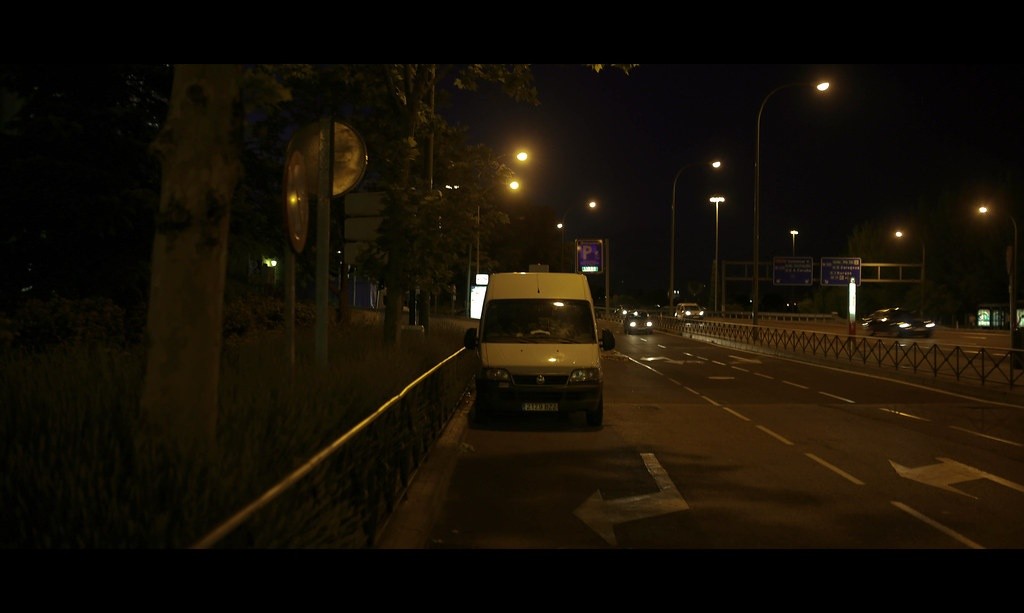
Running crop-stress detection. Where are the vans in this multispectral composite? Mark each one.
[465,274,615,432]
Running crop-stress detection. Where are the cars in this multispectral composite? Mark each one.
[623,311,653,335]
[675,302,705,321]
[862,307,939,338]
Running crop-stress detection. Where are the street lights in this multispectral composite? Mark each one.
[559,198,599,274]
[896,232,902,315]
[790,230,799,257]
[474,150,530,276]
[669,157,725,318]
[751,80,833,338]
[708,193,725,315]
[978,205,1017,318]
